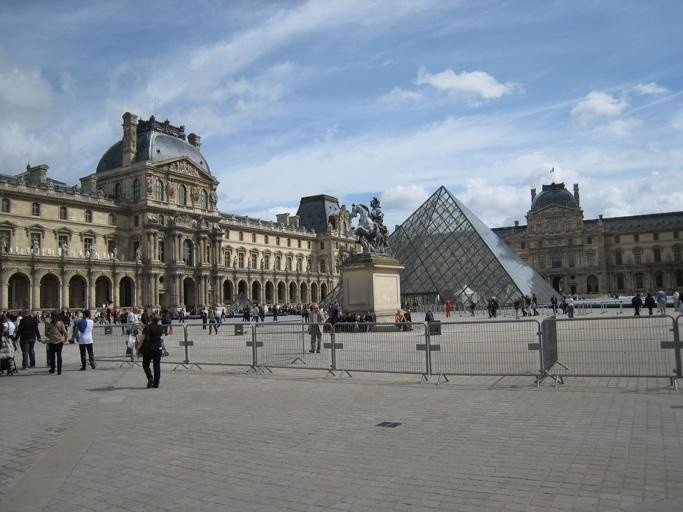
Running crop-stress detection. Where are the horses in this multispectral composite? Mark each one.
[351,204,389,253]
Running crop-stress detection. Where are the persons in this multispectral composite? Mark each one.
[0,300,186,387]
[395,288,683,331]
[355,197,384,243]
[197,301,371,353]
